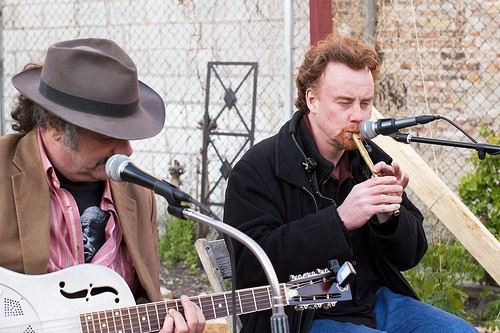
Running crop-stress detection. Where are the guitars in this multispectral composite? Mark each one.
[0,259,357,333]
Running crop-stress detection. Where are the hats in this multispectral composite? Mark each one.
[12,37,167,141]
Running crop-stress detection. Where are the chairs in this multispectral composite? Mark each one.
[194,237,243,333]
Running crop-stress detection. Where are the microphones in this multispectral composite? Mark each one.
[360,114,440,139]
[105,153,193,202]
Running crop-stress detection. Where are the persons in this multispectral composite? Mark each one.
[0,38,205,333]
[223,32,479,333]
